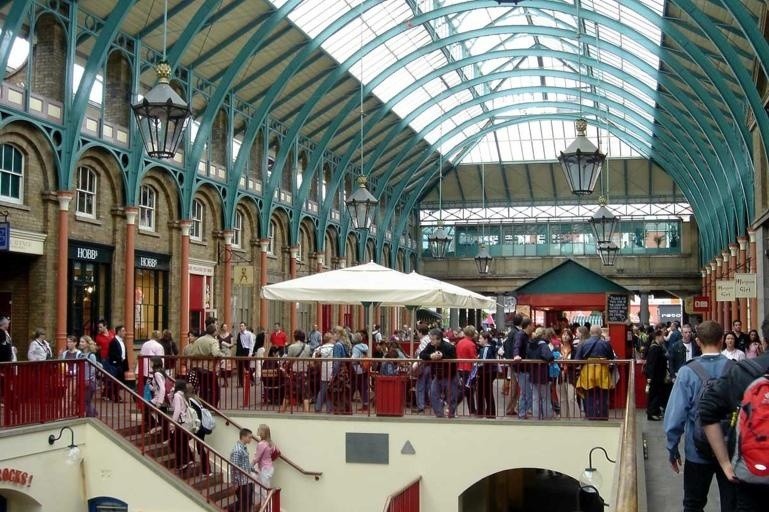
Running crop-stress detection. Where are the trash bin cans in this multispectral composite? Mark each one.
[375,376,408,417]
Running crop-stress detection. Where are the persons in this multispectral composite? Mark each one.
[229,428,260,511]
[185,381,216,476]
[696,316,769,511]
[663,320,737,511]
[143,356,175,444]
[250,424,281,508]
[1,314,767,417]
[167,377,202,470]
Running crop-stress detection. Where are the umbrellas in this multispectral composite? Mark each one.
[261,259,491,389]
[320,270,497,369]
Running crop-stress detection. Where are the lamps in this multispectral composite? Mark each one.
[596,240,620,268]
[427,73,455,262]
[474,162,494,276]
[586,126,621,245]
[47,425,81,466]
[342,1,380,234]
[556,0,608,198]
[579,445,617,494]
[127,0,194,161]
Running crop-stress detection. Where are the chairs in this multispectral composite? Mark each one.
[256,363,433,417]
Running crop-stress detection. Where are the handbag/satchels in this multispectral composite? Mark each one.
[409,357,427,379]
[269,442,279,461]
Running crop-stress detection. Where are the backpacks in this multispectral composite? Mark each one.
[153,372,175,408]
[88,351,105,380]
[355,344,372,370]
[685,357,769,477]
[177,392,215,437]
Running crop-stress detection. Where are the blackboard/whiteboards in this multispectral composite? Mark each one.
[605,292,631,324]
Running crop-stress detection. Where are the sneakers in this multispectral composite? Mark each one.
[236,384,244,389]
[221,382,229,388]
[469,408,498,420]
[505,410,519,417]
[647,414,664,422]
[356,404,370,412]
[100,394,126,404]
[175,460,215,481]
[411,408,425,416]
